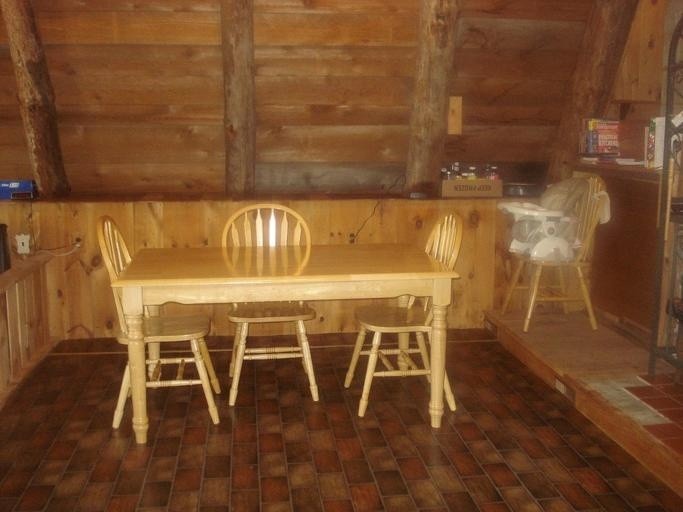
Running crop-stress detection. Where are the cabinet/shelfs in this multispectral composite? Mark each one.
[566,158,682,355]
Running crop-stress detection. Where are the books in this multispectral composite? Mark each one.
[588,118,620,155]
[643,116,665,169]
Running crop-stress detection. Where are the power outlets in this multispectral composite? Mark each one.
[71,232,85,247]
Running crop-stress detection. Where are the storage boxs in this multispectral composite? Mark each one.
[437,180,502,199]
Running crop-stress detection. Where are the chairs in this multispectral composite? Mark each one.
[97,216,222,428]
[222,203,321,406]
[501,174,606,332]
[342,210,463,418]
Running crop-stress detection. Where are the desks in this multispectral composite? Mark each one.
[111,244,461,442]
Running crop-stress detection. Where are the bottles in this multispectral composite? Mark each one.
[440,161,500,181]
